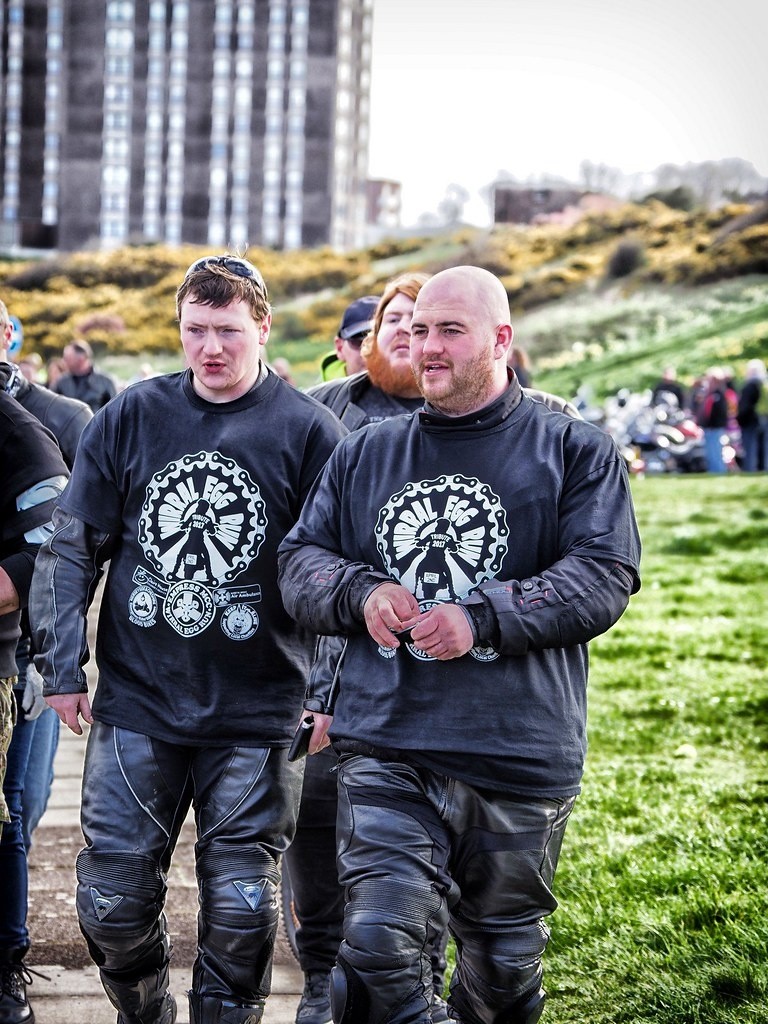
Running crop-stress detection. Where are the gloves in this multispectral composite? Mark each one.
[21,664,53,720]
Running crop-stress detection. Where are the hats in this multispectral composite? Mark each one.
[338,295,383,339]
[7,316,23,362]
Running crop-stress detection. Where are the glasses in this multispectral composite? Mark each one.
[184,255,268,301]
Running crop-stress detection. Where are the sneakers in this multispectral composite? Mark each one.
[295,972,334,1024]
[431,994,456,1024]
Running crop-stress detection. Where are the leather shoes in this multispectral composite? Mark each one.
[0,938,51,1024]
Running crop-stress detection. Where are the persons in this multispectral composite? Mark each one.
[0,300,117,1024]
[275,266,641,1024]
[272,274,584,1023]
[29,256,352,1024]
[650,359,768,473]
